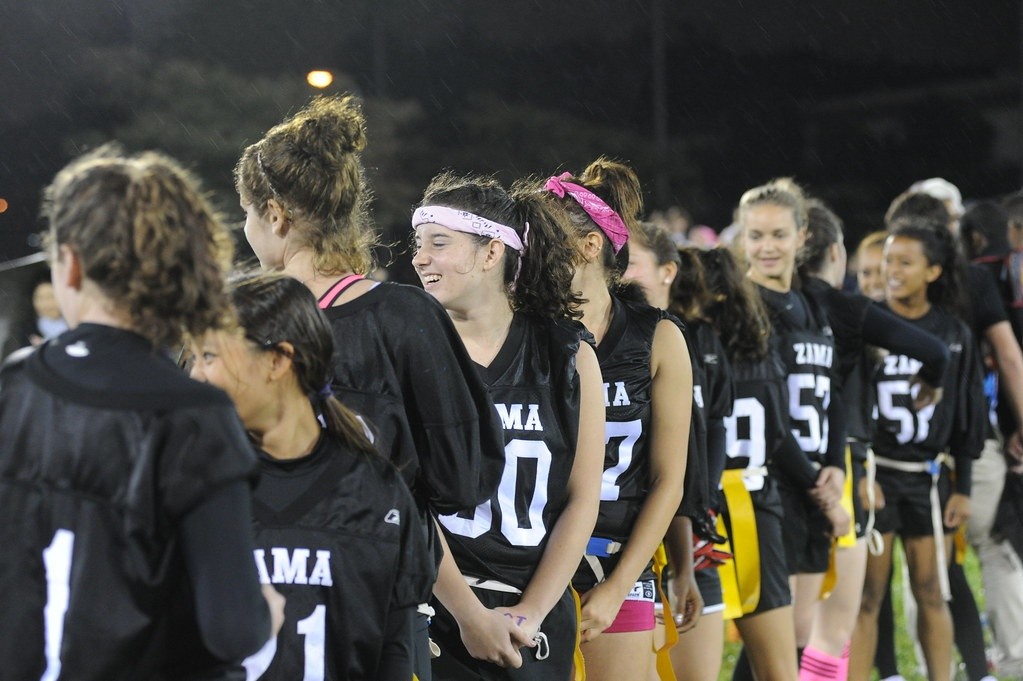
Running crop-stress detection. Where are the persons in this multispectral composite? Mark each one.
[186,275,440,681]
[537,158,692,681]
[410,177,607,681]
[621,177,1023,681]
[234,97,507,681]
[0,153,287,681]
[18,266,69,348]
